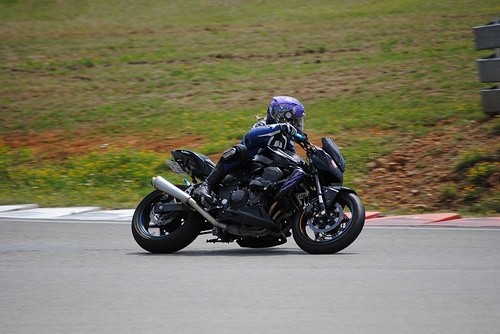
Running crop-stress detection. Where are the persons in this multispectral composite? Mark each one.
[193,96,307,203]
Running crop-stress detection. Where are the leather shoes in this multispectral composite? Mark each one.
[192,181,213,204]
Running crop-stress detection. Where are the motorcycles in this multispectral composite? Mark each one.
[130,128,365,255]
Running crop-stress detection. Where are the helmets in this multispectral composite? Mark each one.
[266,96,305,125]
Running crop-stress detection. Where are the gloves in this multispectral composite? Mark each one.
[280,122,297,141]
